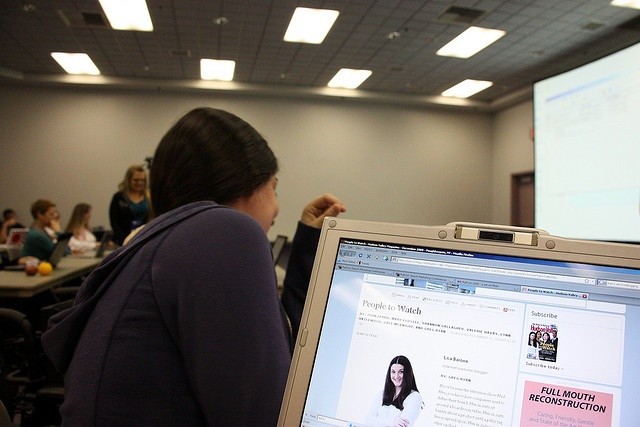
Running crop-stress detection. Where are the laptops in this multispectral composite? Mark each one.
[76,230,111,258]
[271,234,288,261]
[5,231,72,272]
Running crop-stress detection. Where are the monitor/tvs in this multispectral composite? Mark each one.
[274,212,640,426]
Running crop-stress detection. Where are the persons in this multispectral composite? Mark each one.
[368,355,422,426]
[61,108,346,427]
[110,164,154,246]
[526,330,558,363]
[0,208,24,243]
[65,203,116,253]
[25,199,57,262]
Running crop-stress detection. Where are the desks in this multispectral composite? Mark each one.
[1,246,117,301]
